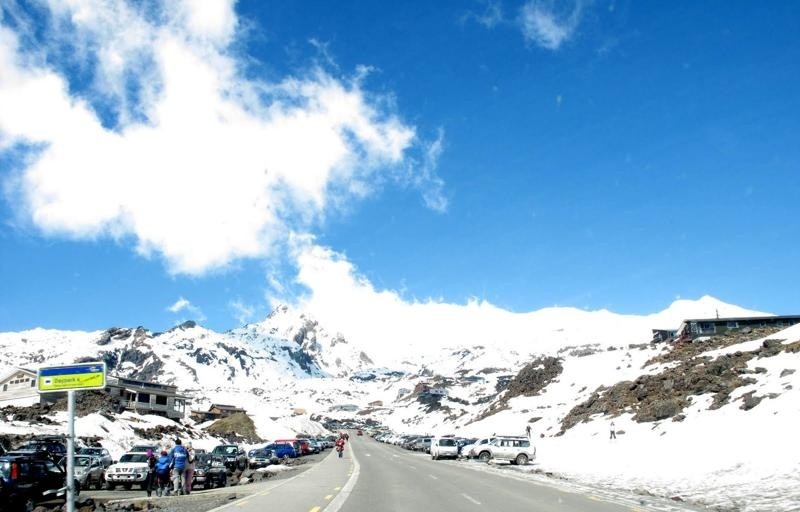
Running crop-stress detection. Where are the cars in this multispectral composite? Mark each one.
[357,430,535,464]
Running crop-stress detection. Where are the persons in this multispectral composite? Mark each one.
[185,441,196,495]
[168,438,194,497]
[608,421,617,440]
[144,449,157,496]
[335,438,345,453]
[154,450,173,497]
[525,425,533,439]
